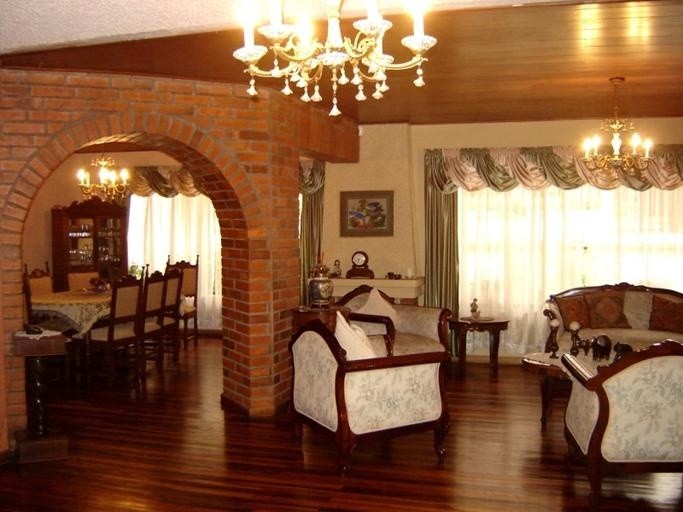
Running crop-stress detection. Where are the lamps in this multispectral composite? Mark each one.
[232,0,439,118]
[76,153,130,203]
[577,76,656,172]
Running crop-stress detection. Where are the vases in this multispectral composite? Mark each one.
[308,264,334,309]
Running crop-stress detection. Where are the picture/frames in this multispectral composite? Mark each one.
[340,190,394,237]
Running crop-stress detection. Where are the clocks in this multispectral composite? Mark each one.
[346,251,374,279]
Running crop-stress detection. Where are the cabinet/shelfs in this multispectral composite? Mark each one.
[50,195,128,291]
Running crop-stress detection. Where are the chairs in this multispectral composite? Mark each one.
[66,269,100,291]
[24,260,53,295]
[86,280,142,386]
[140,270,166,371]
[162,269,185,361]
[166,254,200,347]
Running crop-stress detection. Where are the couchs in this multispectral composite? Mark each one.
[543,283,683,355]
[288,319,451,474]
[561,339,683,503]
[336,285,452,355]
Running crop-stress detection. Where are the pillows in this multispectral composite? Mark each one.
[334,311,377,362]
[350,288,397,335]
